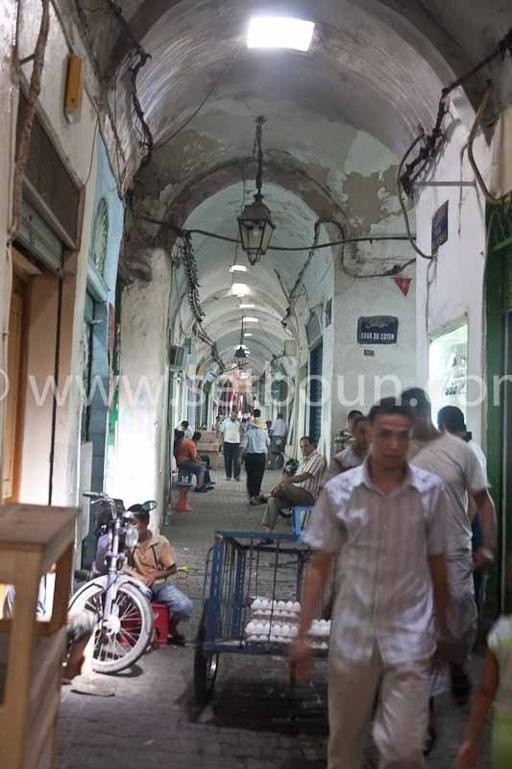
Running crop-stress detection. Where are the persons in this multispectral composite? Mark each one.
[455,612,512,768]
[173,408,288,505]
[317,387,488,645]
[284,396,450,768]
[399,388,498,755]
[255,435,328,545]
[119,503,194,644]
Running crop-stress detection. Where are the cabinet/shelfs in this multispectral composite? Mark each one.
[3,499,84,768]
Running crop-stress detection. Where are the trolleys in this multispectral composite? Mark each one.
[188,527,337,706]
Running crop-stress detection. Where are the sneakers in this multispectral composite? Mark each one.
[225,477,232,481]
[255,492,271,505]
[233,476,240,482]
[195,479,216,494]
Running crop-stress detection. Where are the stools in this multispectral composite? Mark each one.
[175,481,193,511]
[291,507,313,535]
[119,601,171,645]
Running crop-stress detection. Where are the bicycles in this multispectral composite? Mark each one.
[270,450,299,520]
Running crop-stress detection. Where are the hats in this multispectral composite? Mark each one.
[249,418,269,429]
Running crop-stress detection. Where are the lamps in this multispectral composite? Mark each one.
[234,314,247,370]
[235,117,277,265]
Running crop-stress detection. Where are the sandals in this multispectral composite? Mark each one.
[255,537,276,544]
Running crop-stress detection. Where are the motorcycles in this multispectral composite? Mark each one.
[66,489,157,674]
